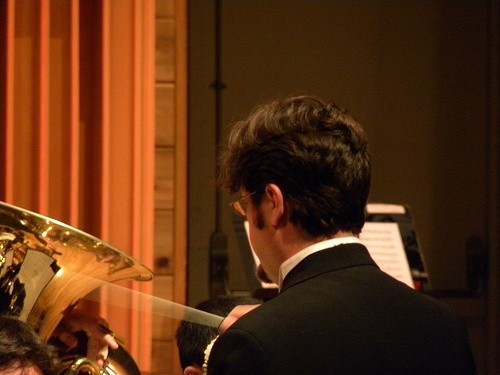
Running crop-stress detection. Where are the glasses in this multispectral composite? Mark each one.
[228,191,259,218]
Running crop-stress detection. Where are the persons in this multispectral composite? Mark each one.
[206,95,478,375]
[176,295,264,375]
[0,309,119,375]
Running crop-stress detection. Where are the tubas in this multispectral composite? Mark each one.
[2,199,153,370]
